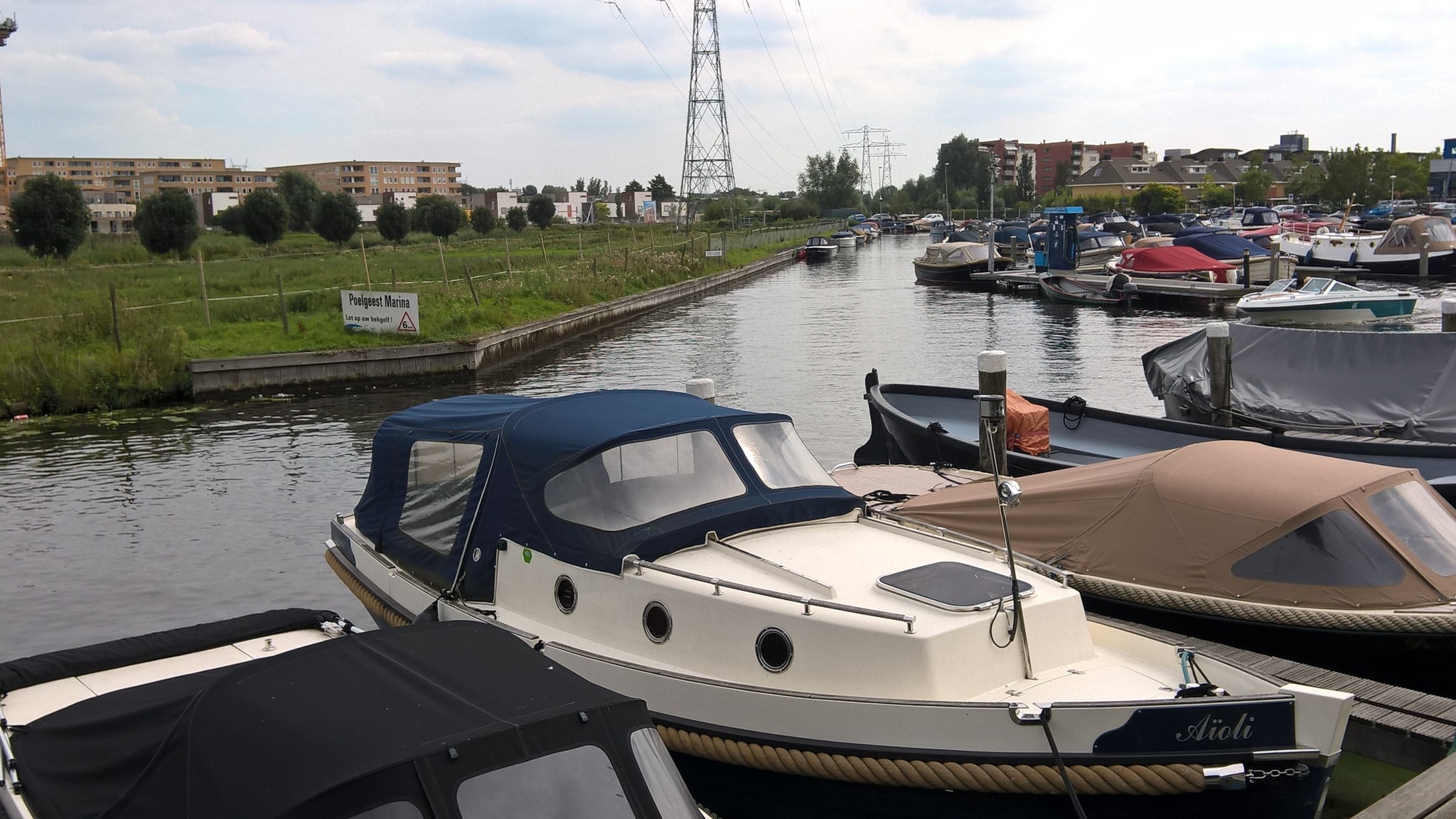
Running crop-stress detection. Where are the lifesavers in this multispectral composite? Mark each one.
[1316,226,1329,234]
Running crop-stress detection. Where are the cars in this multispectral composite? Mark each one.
[920,214,945,223]
[1362,198,1456,220]
[1294,203,1333,215]
[870,213,892,221]
[1271,205,1296,217]
[853,214,866,221]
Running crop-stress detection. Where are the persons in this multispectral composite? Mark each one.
[1284,276,1319,292]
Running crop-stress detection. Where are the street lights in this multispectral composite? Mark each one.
[1390,174,1396,207]
[879,195,883,224]
[1231,182,1237,208]
[977,146,1001,274]
[945,163,950,222]
[878,167,882,190]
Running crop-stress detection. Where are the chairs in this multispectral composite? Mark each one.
[602,473,699,530]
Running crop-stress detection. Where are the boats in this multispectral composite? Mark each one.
[931,207,1456,286]
[852,218,883,244]
[1039,272,1141,306]
[0,606,711,819]
[804,236,839,258]
[1275,215,1456,285]
[851,369,1456,520]
[824,438,1456,700]
[912,242,1014,284]
[830,228,857,247]
[1235,277,1419,326]
[1105,246,1238,284]
[322,388,1358,819]
[1140,319,1456,458]
